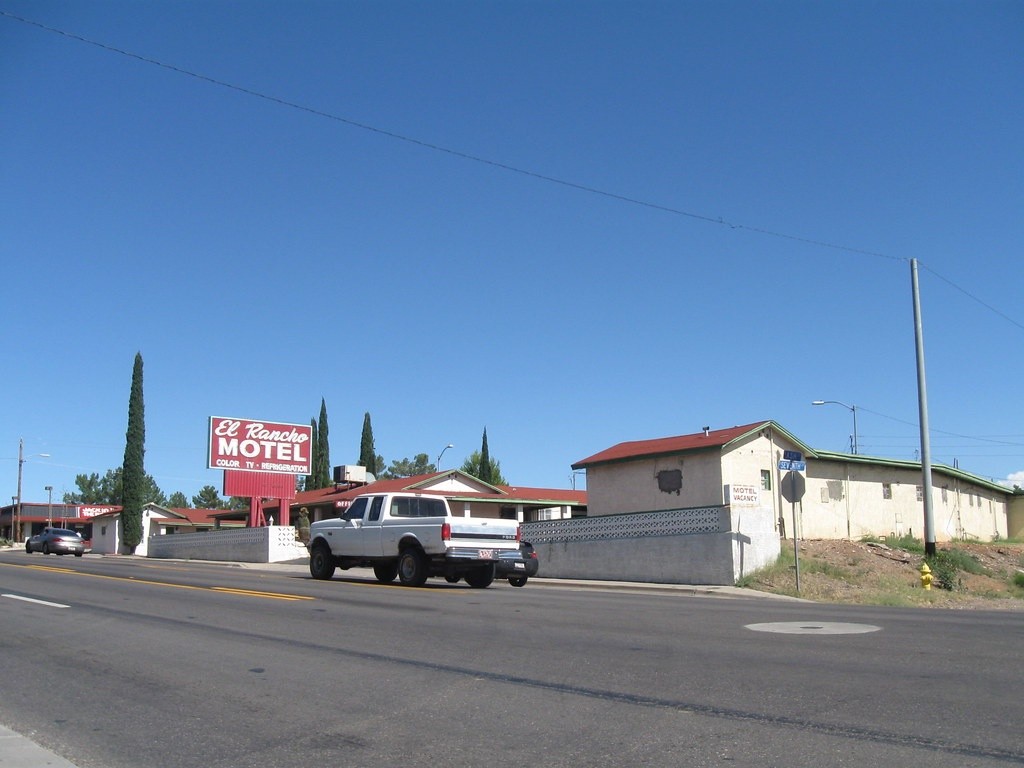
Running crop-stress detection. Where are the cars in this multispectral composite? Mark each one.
[25,528,85,558]
[372,540,539,588]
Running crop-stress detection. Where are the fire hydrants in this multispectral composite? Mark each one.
[920,562,935,592]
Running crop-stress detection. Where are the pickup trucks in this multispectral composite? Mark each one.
[306,492,526,588]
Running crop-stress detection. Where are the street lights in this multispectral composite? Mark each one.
[11,496,20,542]
[811,399,860,455]
[44,486,53,528]
[15,453,51,544]
[437,444,454,473]
